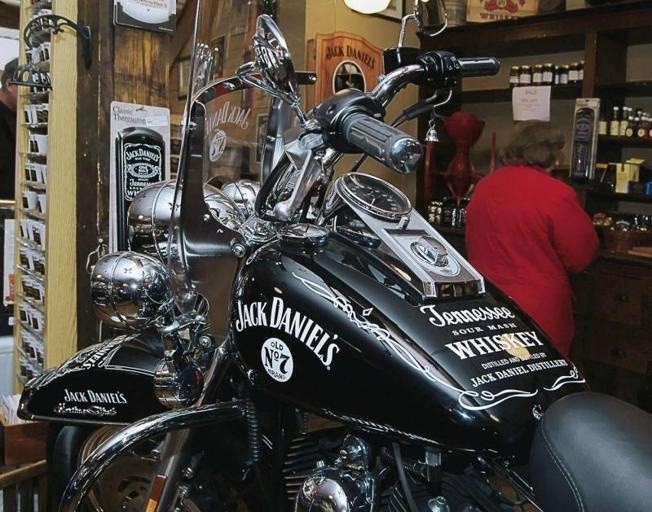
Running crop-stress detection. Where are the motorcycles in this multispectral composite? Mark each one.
[16,0,652,512]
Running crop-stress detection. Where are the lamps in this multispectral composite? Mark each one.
[343,0,390,15]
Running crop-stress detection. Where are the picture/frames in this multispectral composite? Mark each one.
[113,0,176,35]
[177,55,190,99]
[314,32,384,110]
[208,32,231,82]
[351,0,406,24]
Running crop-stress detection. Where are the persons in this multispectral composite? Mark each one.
[0,53,33,306]
[467,121,600,364]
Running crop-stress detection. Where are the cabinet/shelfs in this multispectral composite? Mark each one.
[441,234,584,385]
[421,8,583,235]
[584,0,651,238]
[13,0,96,396]
[586,252,651,414]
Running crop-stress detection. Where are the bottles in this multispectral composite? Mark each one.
[598,106,652,137]
[509,61,585,85]
